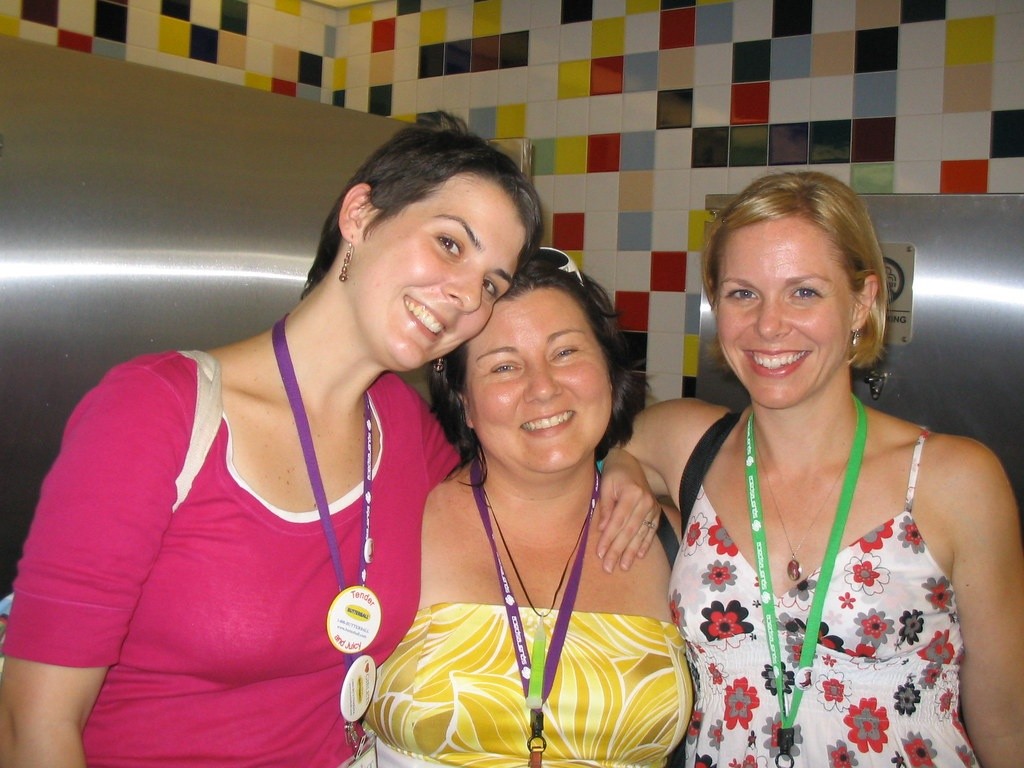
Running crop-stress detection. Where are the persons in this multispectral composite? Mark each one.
[0,117,1024,768]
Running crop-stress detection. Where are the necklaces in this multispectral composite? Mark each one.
[753,431,849,581]
[483,461,597,709]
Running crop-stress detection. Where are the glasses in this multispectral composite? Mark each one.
[538,246,583,283]
